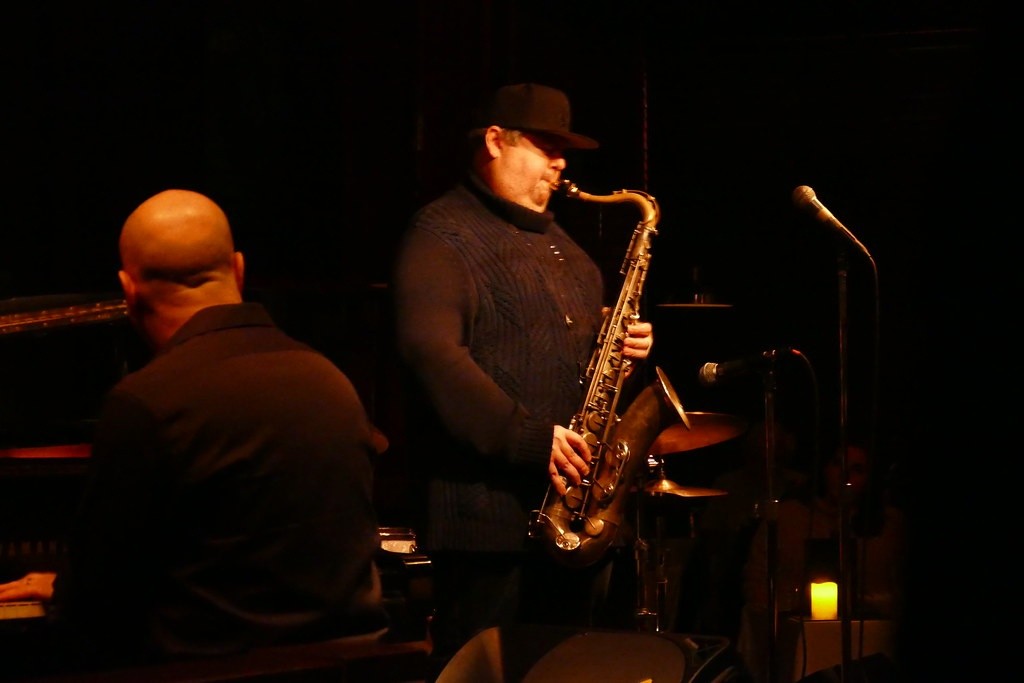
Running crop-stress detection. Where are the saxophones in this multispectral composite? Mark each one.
[528,179,692,560]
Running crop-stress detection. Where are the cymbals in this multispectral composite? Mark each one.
[628,467,729,498]
[646,411,747,456]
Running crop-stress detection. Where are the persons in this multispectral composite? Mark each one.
[398,80,652,683]
[2,188,376,683]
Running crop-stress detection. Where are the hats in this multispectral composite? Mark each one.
[468,85,599,149]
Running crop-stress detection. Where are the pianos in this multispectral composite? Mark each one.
[0,291,438,683]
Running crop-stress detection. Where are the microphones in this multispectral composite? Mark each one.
[791,186,872,260]
[699,347,794,386]
